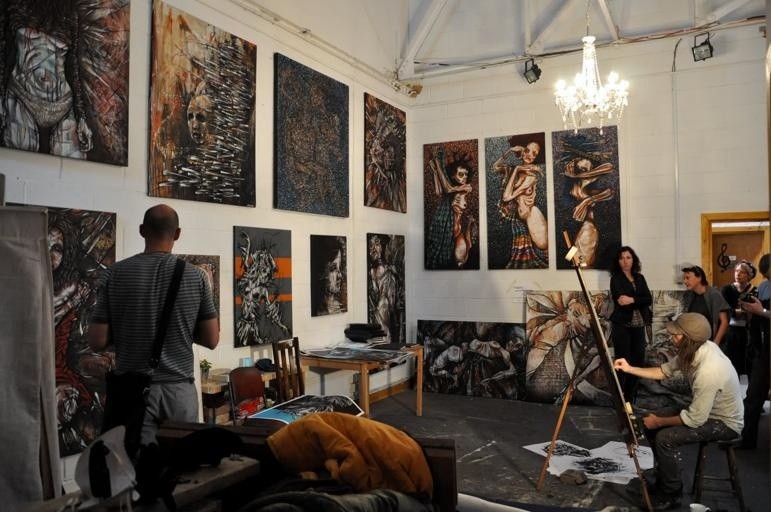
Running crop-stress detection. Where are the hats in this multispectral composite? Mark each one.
[666,312,711,344]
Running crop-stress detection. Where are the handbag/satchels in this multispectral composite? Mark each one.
[101,367,152,462]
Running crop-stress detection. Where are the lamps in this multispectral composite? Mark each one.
[550,0,633,137]
[523,58,543,86]
[691,30,714,62]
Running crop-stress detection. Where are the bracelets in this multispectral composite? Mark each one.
[762,307,768,318]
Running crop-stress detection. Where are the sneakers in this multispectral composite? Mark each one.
[731,436,757,448]
[649,494,682,512]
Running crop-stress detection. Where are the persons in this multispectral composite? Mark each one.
[612,310,749,511]
[608,244,657,407]
[738,296,771,320]
[680,263,732,349]
[759,253,771,452]
[83,201,222,446]
[721,258,758,375]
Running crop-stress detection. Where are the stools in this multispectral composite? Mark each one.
[689,433,749,512]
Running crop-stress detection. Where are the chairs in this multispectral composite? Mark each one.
[227,364,268,426]
[272,338,304,410]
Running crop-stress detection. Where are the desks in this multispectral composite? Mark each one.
[200,360,305,428]
[298,339,427,424]
